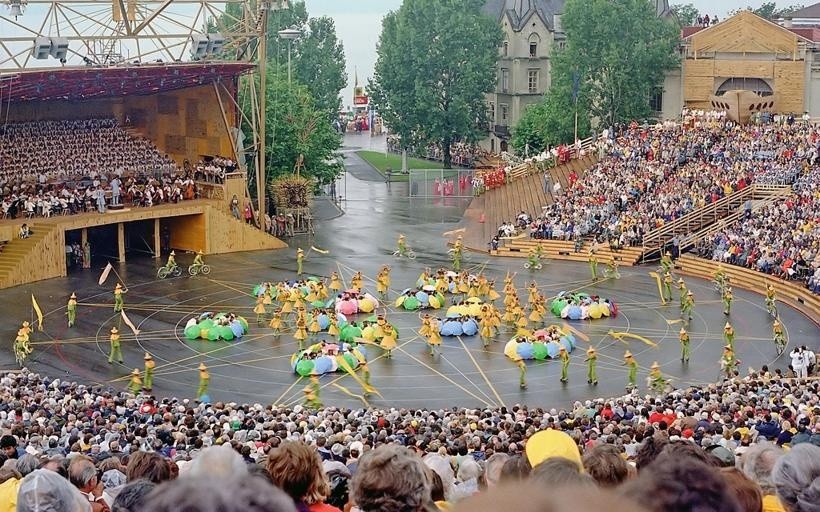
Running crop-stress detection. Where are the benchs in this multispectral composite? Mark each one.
[484,153,600,243]
[647,195,751,249]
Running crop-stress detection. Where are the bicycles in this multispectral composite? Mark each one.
[447,248,471,260]
[158,264,182,278]
[645,382,672,395]
[774,335,785,353]
[392,247,415,260]
[523,257,542,270]
[601,264,621,279]
[766,301,778,318]
[718,363,739,379]
[187,264,211,275]
[14,349,26,367]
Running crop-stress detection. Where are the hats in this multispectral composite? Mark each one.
[1,235,819,512]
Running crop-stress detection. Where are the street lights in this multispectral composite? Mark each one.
[280,24,300,88]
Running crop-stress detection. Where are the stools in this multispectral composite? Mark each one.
[3,192,114,219]
